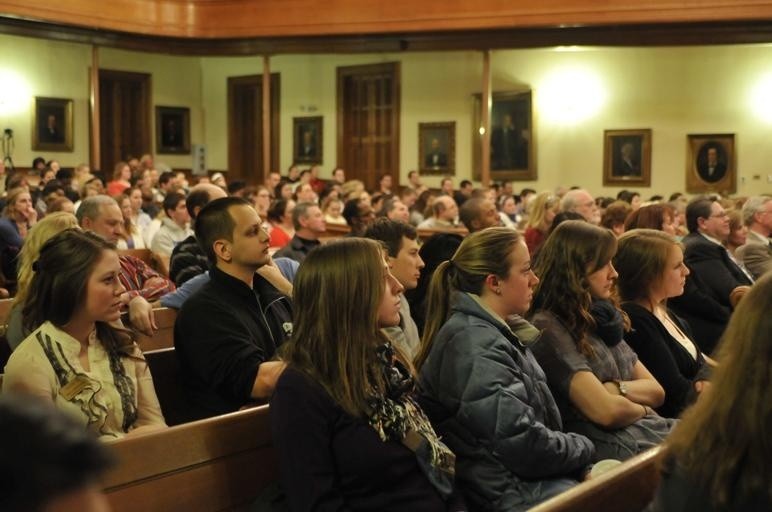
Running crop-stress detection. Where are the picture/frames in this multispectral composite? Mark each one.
[602,127,652,189]
[471,87,536,183]
[155,104,191,155]
[292,115,324,165]
[30,95,74,152]
[685,133,736,196]
[418,121,456,177]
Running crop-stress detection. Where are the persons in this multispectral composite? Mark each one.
[426,138,448,166]
[0,378,115,511]
[614,143,640,175]
[264,237,498,511]
[0,154,771,443]
[164,119,182,146]
[413,227,595,512]
[700,147,726,183]
[40,114,63,143]
[491,115,520,167]
[648,273,772,511]
[526,221,685,464]
[300,131,316,156]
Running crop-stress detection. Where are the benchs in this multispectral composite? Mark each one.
[1,220,665,509]
